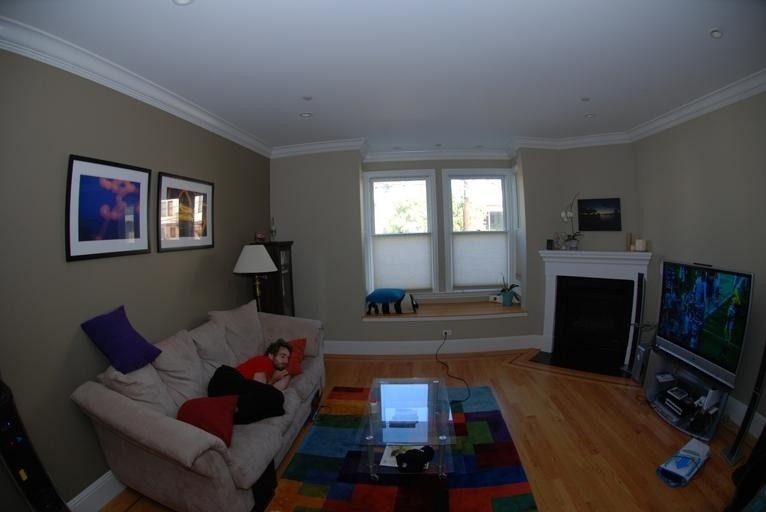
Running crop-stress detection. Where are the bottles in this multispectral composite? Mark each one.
[269,217,277,241]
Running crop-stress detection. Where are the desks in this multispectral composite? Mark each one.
[642,346,733,445]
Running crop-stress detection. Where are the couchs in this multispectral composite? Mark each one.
[68,312,326,512]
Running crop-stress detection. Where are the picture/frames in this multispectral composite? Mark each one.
[65,153,152,263]
[157,171,215,253]
[573,194,625,233]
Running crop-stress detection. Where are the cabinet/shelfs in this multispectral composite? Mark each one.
[256,241,295,318]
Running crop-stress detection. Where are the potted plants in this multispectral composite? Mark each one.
[492,276,521,306]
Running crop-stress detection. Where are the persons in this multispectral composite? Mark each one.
[660,263,744,356]
[206,336,292,426]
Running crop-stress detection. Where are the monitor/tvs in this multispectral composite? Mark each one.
[652,259,755,391]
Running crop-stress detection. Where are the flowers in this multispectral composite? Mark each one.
[561,192,584,239]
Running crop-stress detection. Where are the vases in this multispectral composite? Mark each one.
[566,238,578,249]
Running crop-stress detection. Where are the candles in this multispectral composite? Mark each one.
[636,237,646,250]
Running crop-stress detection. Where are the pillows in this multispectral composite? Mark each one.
[79,299,307,448]
[366,288,406,303]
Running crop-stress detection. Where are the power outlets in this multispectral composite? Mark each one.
[443,330,452,336]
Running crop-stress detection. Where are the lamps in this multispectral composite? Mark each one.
[232,244,278,312]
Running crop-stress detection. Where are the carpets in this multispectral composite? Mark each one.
[264,384,539,512]
